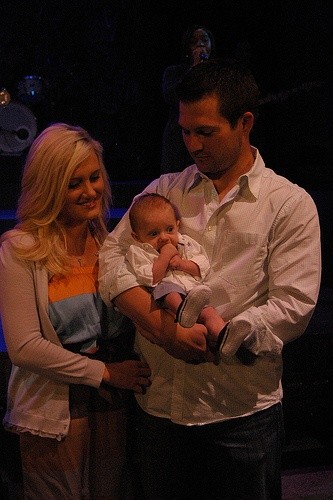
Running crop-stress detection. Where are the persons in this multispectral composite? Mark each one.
[0,121,150,500]
[159,24,219,176]
[97,56,322,500]
[124,191,249,361]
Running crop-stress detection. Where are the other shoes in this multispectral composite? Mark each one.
[216,320,252,359]
[176,285,212,328]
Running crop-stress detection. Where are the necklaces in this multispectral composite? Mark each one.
[51,237,91,265]
